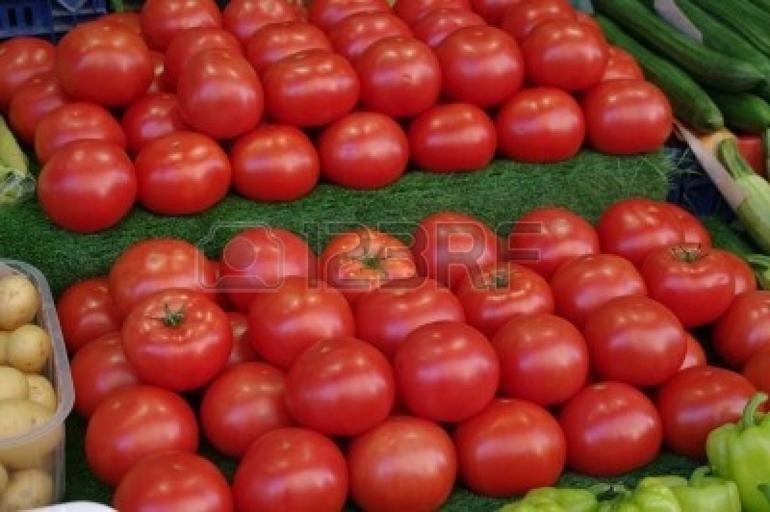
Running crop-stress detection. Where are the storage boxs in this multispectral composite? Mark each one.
[0,258,76,511]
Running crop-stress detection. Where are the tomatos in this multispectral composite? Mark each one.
[0,0,770,512]
